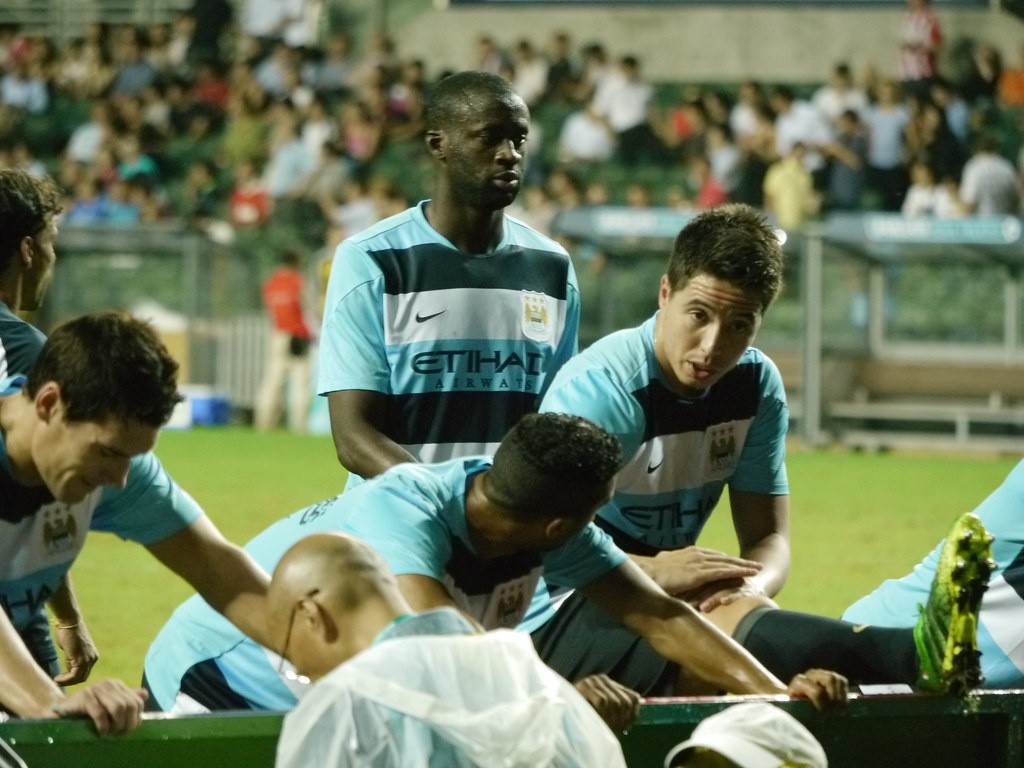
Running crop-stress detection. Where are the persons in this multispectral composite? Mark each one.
[134,409,851,712]
[1,310,279,737]
[263,532,629,768]
[1,164,98,693]
[508,212,994,700]
[664,696,832,768]
[836,459,1024,700]
[313,71,585,496]
[1,2,1024,428]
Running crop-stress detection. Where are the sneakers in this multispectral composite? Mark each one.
[913,514,996,700]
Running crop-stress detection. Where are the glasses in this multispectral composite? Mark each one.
[277,589,310,687]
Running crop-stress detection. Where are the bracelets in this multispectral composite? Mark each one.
[56,618,83,630]
